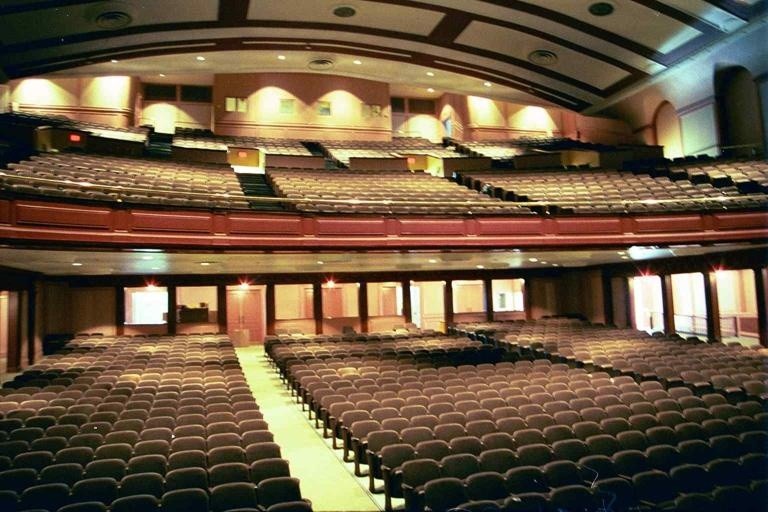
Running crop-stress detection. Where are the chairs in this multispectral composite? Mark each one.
[0,110,768,511]
